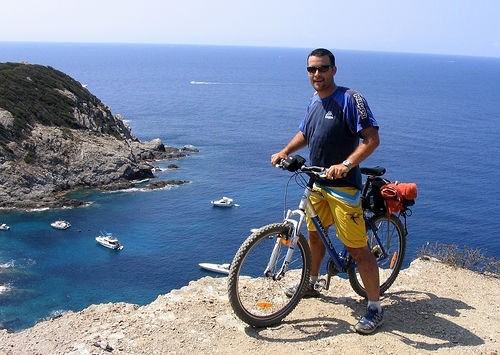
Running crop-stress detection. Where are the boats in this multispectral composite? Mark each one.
[198,262,230,275]
[0,223,11,231]
[50,219,72,230]
[210,196,240,207]
[95,230,124,251]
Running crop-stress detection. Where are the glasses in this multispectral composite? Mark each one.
[306,65,331,73]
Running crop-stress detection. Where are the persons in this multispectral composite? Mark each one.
[270,48,385,335]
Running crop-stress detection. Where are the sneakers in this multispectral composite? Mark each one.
[354,305,384,333]
[286,281,319,298]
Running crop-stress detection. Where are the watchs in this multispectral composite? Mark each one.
[343,160,352,170]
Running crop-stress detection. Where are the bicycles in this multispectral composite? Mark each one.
[227,154,416,329]
